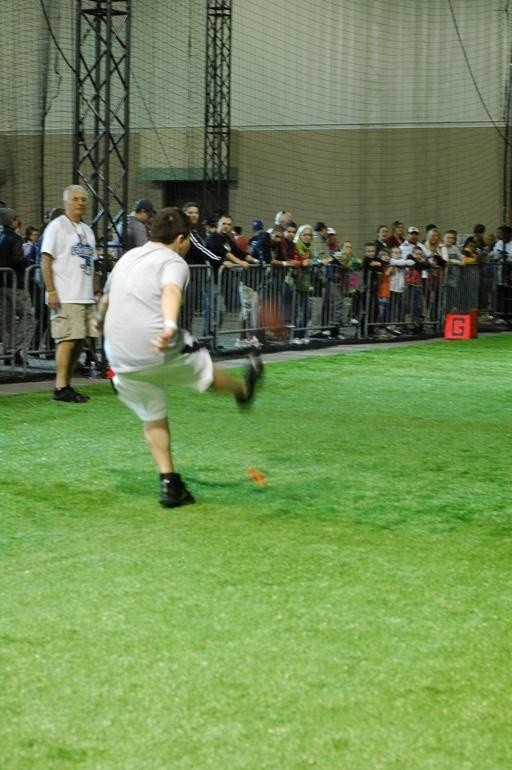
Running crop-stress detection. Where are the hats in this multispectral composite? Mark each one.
[252,220,263,229]
[135,199,156,218]
[407,226,419,233]
[326,227,336,235]
[0,207,16,227]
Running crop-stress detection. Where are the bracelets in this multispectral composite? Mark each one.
[282,261,287,267]
[300,260,303,267]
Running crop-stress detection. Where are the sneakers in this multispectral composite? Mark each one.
[234,338,252,348]
[234,353,263,406]
[68,384,89,400]
[290,326,402,346]
[53,389,86,403]
[158,478,194,507]
[247,335,264,347]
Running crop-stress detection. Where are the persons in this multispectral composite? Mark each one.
[1,202,65,364]
[235,211,361,348]
[91,206,263,507]
[177,205,233,341]
[355,222,511,340]
[115,202,155,263]
[230,225,248,253]
[40,184,101,403]
[200,216,260,349]
